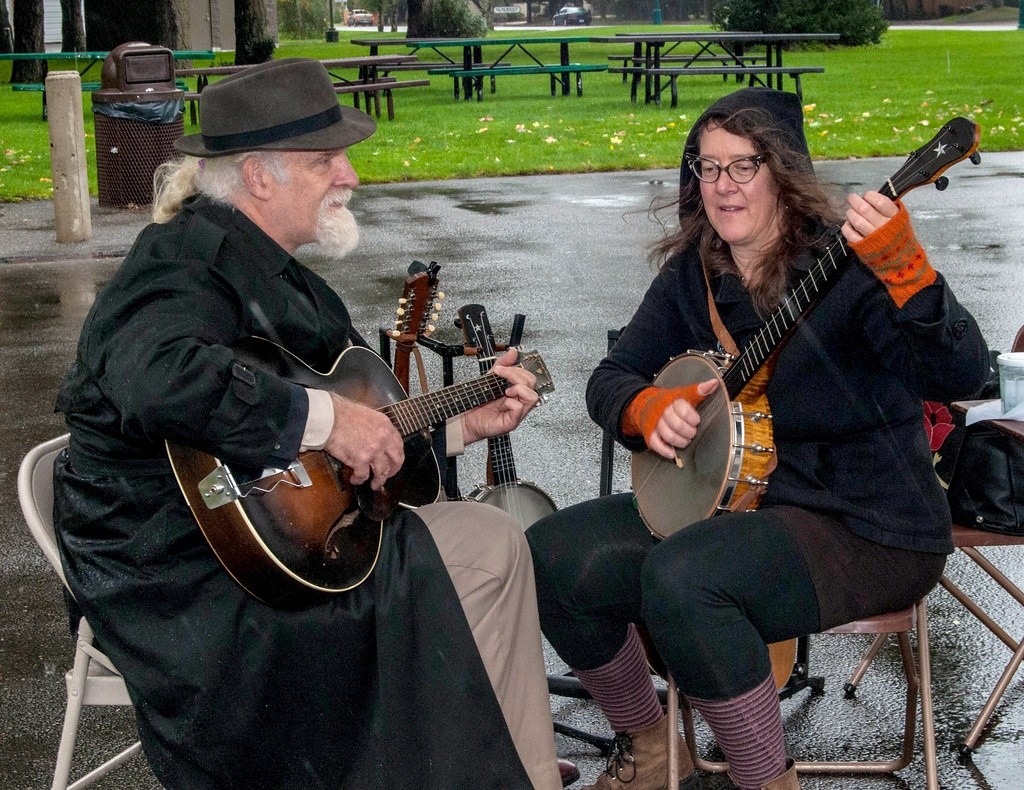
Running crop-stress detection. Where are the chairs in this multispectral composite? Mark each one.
[16,433,150,790]
[843,323,1024,756]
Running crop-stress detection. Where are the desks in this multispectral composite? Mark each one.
[0,30,840,107]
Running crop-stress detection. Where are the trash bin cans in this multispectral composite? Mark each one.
[90,41,185,210]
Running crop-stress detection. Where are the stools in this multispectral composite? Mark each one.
[666,595,939,790]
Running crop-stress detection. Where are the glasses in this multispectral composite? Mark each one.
[683,153,772,184]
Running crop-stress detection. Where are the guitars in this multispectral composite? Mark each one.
[392,256,449,396]
[161,345,555,612]
[448,302,561,541]
[628,114,988,541]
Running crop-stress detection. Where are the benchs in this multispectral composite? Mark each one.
[10,53,825,122]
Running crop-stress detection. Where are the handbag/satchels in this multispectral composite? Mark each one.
[945,420,1024,536]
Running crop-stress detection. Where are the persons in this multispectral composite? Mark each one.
[55,65,580,790]
[524,89,991,790]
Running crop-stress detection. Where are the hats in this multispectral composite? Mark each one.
[176,58,376,158]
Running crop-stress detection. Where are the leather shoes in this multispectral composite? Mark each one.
[554,757,580,786]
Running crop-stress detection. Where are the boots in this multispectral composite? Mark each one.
[728,756,801,790]
[583,706,699,790]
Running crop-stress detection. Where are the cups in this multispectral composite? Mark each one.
[996,352,1024,415]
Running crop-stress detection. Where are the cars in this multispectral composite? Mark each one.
[347,8,375,27]
[552,6,590,25]
